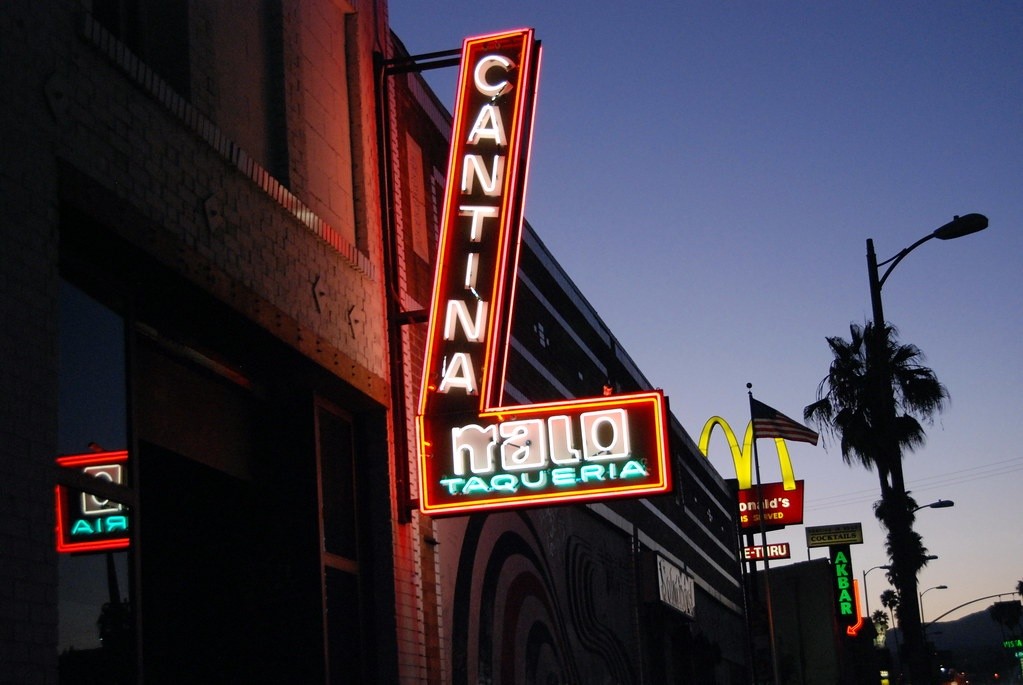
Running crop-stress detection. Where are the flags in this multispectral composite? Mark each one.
[749,395,818,445]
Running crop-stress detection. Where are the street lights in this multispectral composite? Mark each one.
[919,586,948,685]
[864,213,990,685]
[863,564,891,617]
